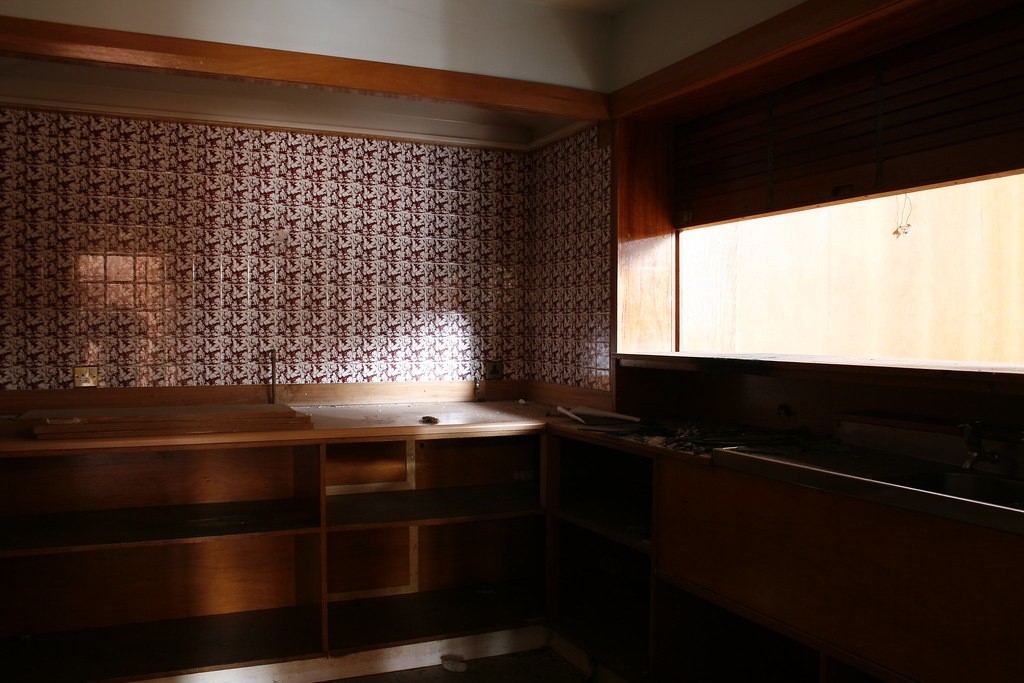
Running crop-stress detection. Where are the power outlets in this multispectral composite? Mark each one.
[74,366,97,387]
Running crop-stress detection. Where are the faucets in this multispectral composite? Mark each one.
[953,417,1000,471]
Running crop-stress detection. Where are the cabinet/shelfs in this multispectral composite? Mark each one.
[547,354,1024,683]
[0,374,613,683]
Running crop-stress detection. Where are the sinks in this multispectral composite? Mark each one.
[872,441,1024,537]
